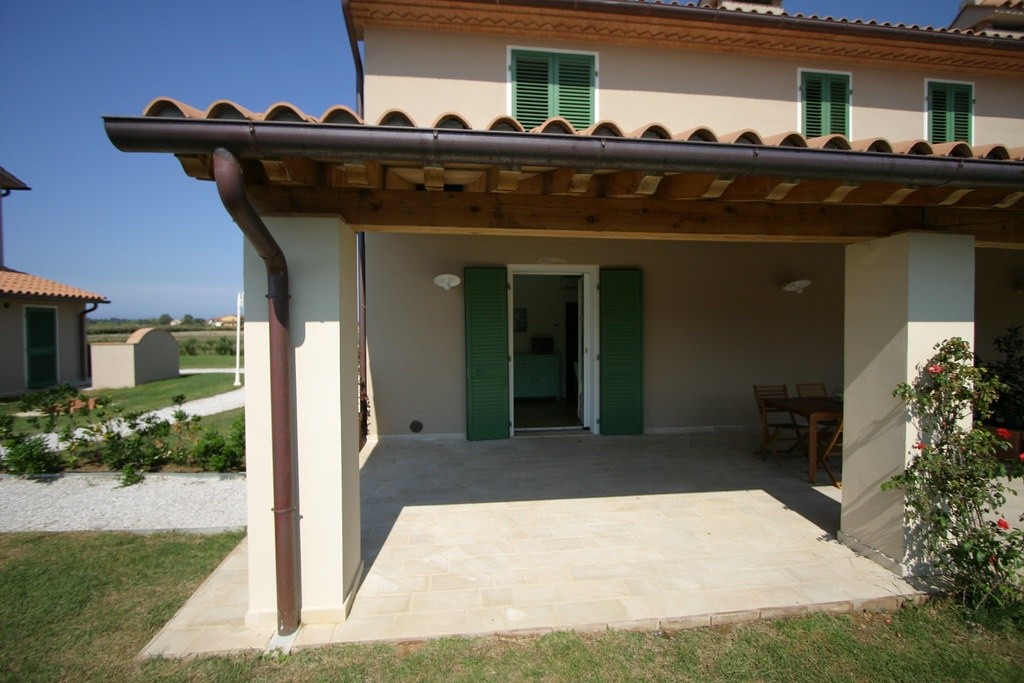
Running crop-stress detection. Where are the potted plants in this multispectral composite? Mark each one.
[974,326,1024,464]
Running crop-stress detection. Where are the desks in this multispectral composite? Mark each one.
[762,397,843,485]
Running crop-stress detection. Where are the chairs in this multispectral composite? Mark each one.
[753,383,843,488]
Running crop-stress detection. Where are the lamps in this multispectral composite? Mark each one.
[433,274,460,290]
